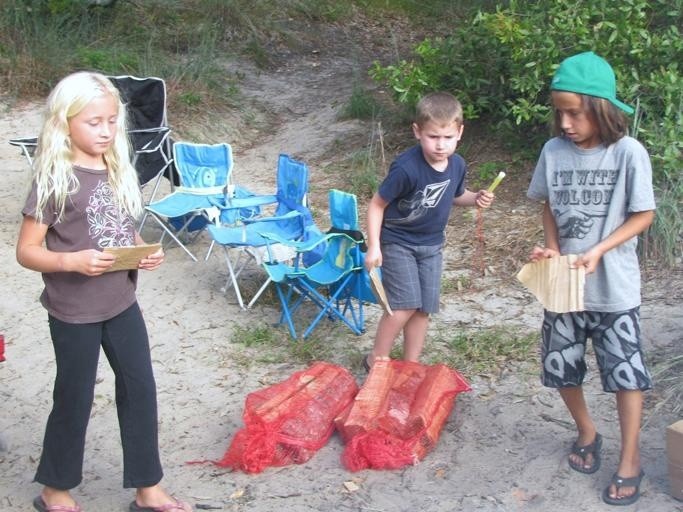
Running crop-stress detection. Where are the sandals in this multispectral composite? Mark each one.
[33,495,82,512]
[130,497,193,512]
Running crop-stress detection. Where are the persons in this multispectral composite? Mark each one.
[527,51,658,506]
[16,70,199,510]
[363,91,495,374]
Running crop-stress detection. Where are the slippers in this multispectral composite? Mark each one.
[603,470,644,506]
[569,432,602,474]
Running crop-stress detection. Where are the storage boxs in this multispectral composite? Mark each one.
[665,419,682,502]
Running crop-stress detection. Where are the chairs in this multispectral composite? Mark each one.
[9,74,181,192]
[204,154,329,308]
[138,141,260,261]
[257,190,382,343]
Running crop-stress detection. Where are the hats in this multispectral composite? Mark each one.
[550,52,634,115]
